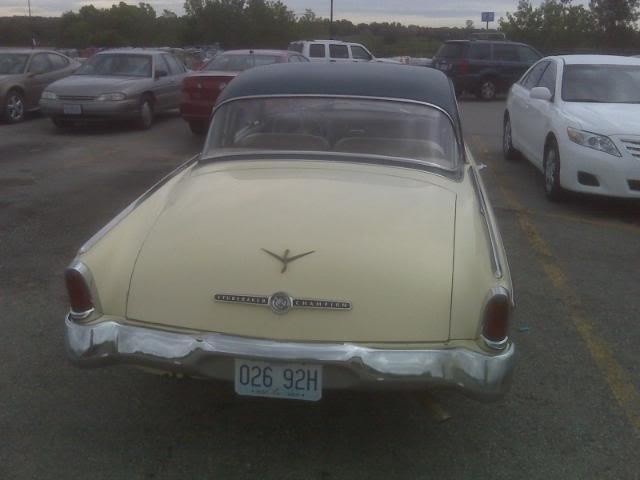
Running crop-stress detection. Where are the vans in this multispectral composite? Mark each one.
[432,40,544,100]
[288,40,375,62]
